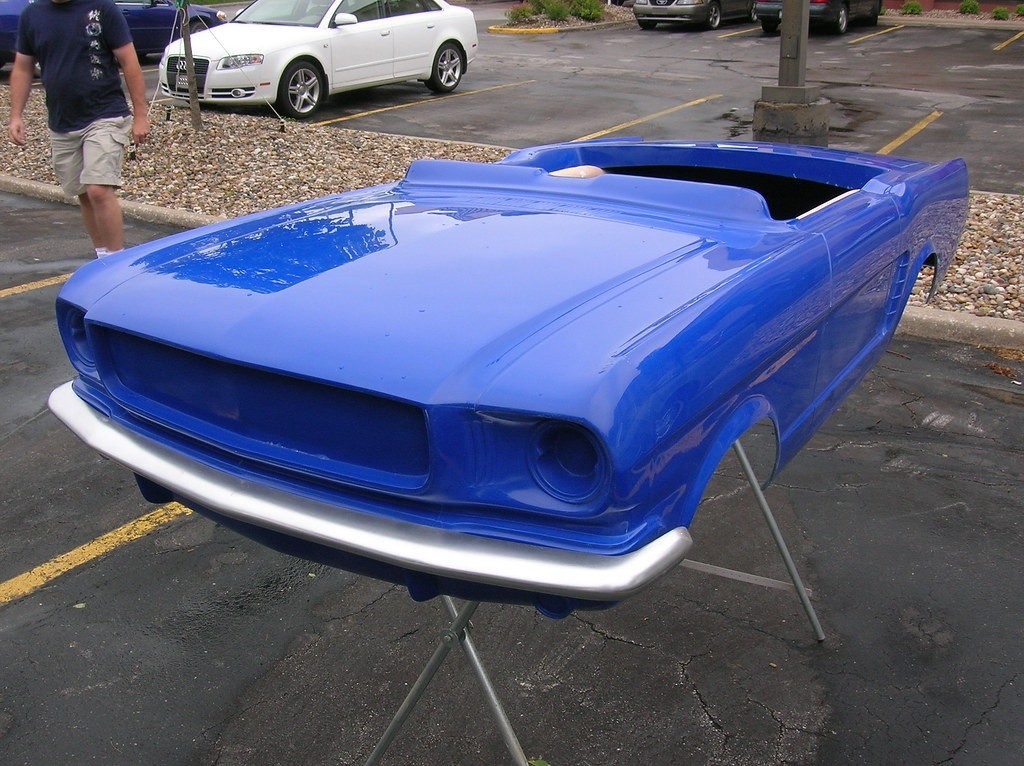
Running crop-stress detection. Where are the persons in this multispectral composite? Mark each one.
[8,0,151,260]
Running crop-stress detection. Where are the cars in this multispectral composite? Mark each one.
[632,0,757,29]
[754,0,884,33]
[114,0,228,62]
[157,0,479,121]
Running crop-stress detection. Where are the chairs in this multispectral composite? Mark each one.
[302,0,348,16]
